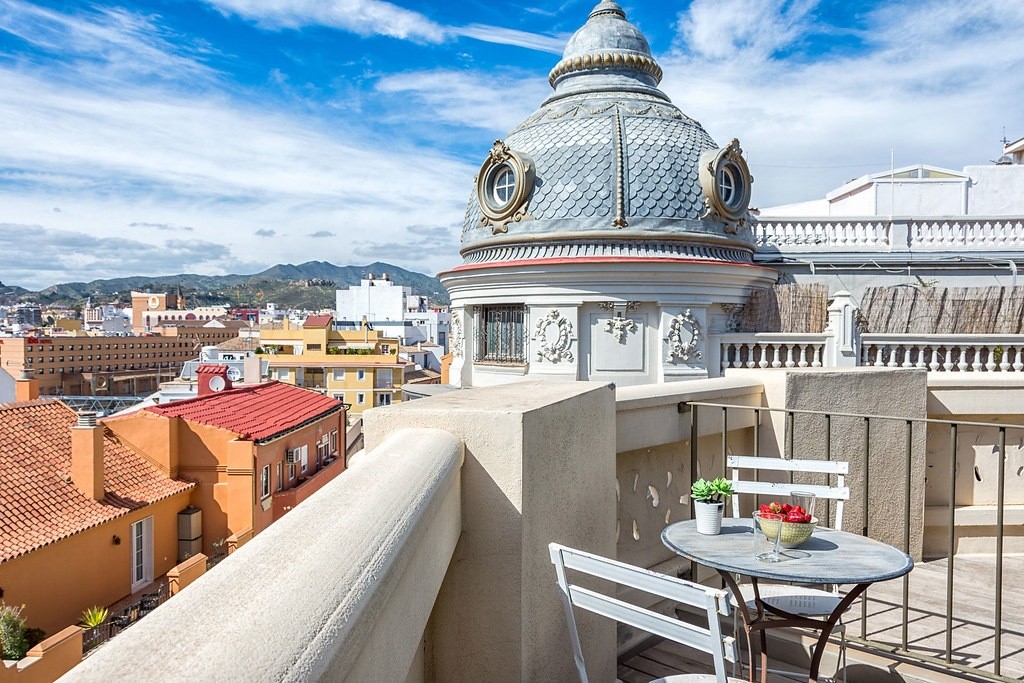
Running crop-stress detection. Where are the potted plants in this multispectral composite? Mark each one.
[691,477,734,535]
[269,344,276,354]
[78,605,109,636]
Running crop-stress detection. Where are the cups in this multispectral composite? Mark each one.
[754,513,782,563]
[790,491,816,517]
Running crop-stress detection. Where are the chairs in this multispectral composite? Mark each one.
[548,542,748,683]
[722,455,851,683]
[109,593,159,638]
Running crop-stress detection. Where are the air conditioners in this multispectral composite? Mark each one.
[288,447,300,464]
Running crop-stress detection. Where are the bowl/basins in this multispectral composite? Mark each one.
[752,510,818,548]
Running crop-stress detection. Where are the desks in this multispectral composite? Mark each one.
[662,518,914,683]
